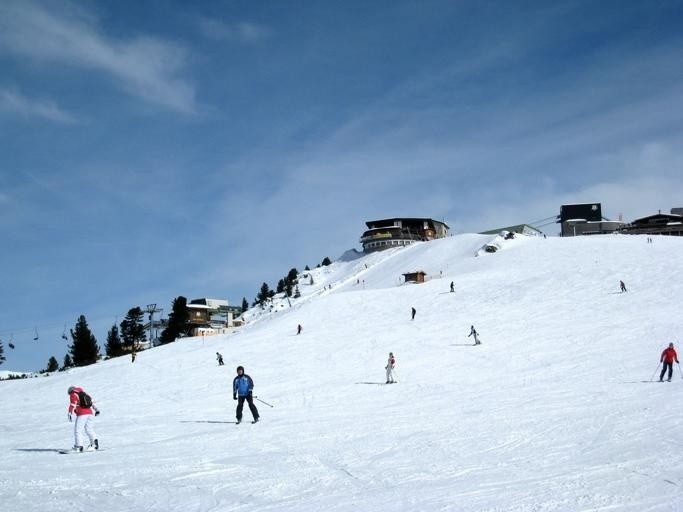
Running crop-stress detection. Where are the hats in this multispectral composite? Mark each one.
[669,343,673,346]
[389,352,393,356]
[68,385,77,394]
[237,366,244,374]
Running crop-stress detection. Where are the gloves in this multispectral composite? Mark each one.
[248,391,252,397]
[660,360,663,363]
[95,410,100,416]
[676,360,680,363]
[68,413,73,422]
[233,393,237,400]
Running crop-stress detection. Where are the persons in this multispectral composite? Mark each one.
[214,351,224,366]
[383,351,396,384]
[130,349,136,363]
[410,307,416,320]
[296,324,302,336]
[448,280,455,292]
[232,365,259,423]
[618,280,626,293]
[66,384,101,452]
[467,324,481,345]
[660,342,680,383]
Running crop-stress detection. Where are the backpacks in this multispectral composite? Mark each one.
[79,392,92,408]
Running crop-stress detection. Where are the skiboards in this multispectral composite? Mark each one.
[60,449,105,453]
[473,343,482,345]
[384,382,398,384]
[236,421,259,424]
[646,381,674,384]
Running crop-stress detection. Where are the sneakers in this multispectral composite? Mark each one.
[386,381,393,384]
[72,446,83,452]
[252,418,258,423]
[236,419,241,424]
[660,377,672,382]
[90,438,99,449]
